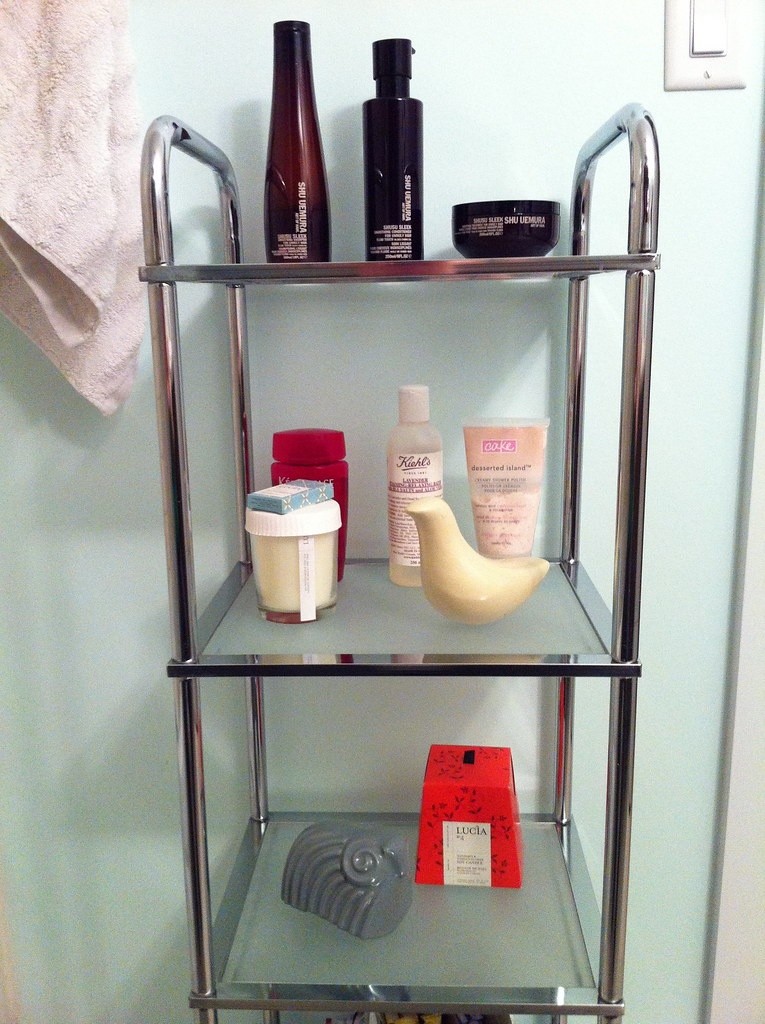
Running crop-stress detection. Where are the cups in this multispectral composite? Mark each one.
[244,501,342,623]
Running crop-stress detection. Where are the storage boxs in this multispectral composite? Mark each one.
[414,744,524,890]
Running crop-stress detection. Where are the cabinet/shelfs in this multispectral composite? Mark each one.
[141,102,664,1024]
